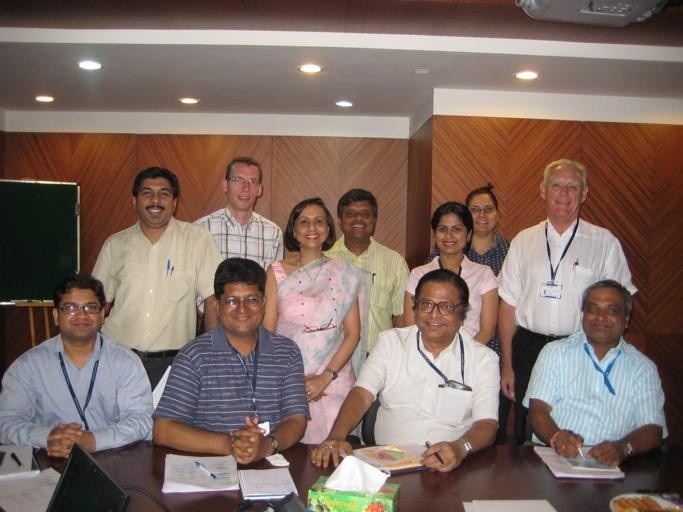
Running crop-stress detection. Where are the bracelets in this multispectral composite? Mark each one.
[548,429,563,447]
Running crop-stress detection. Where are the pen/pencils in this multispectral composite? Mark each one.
[426,442,444,465]
[578,447,585,462]
[11,453,23,468]
[195,461,216,479]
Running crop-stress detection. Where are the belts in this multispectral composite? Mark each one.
[132,348,178,358]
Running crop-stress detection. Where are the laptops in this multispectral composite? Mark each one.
[46,443,130,512]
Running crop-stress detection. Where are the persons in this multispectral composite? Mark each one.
[403,202,498,347]
[422,183,512,443]
[310,268,500,473]
[91,166,223,392]
[263,195,371,443]
[324,188,411,359]
[0,275,152,458]
[192,156,284,313]
[498,158,635,439]
[521,277,669,462]
[150,257,311,465]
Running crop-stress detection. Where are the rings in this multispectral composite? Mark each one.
[329,445,336,449]
[323,446,329,451]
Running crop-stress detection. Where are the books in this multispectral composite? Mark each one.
[531,445,626,480]
[161,454,240,493]
[237,468,298,501]
[350,445,426,476]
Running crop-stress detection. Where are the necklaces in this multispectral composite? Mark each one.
[303,256,322,286]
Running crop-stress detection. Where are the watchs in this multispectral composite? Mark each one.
[459,436,470,457]
[623,439,633,457]
[324,368,337,379]
[269,435,278,454]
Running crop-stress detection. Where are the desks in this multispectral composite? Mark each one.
[30,437,681,512]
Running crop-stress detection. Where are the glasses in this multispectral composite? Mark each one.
[228,177,259,184]
[469,207,495,214]
[304,318,335,333]
[58,302,102,315]
[220,296,261,306]
[415,299,468,314]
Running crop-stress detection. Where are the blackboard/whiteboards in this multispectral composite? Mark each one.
[0,179,80,306]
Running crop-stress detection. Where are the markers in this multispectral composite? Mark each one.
[10,299,41,302]
[43,300,54,303]
